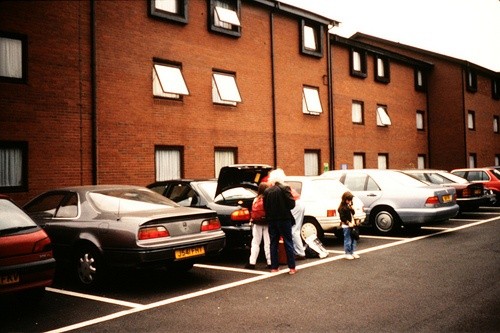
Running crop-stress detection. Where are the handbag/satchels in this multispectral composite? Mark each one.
[352,229,361,241]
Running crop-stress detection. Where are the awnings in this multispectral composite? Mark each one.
[376,107,391,125]
[215,6,242,27]
[154,64,243,103]
[303,88,323,114]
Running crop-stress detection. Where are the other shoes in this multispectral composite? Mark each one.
[265,265,272,269]
[290,268,297,275]
[270,268,279,273]
[244,264,256,270]
[343,254,360,259]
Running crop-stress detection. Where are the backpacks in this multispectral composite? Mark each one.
[251,196,266,220]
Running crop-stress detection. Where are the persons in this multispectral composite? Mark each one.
[247,181,273,268]
[264,168,296,275]
[338,192,361,259]
[261,170,306,261]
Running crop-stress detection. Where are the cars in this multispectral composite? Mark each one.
[318,168,458,234]
[282,174,367,243]
[146,163,277,262]
[1,194,55,308]
[451,166,500,206]
[399,170,485,217]
[19,184,226,287]
